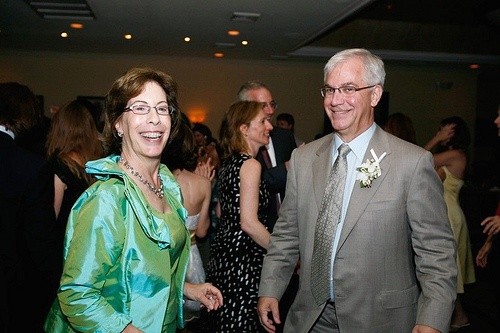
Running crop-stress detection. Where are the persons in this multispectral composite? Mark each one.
[201,99,302,333]
[383,112,416,143]
[422,116,476,329]
[238,81,326,219]
[0,82,222,333]
[476,107,500,333]
[257,47,458,333]
[43,66,223,333]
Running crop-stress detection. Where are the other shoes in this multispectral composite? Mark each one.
[451,322,471,328]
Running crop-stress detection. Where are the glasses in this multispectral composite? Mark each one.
[260,102,278,110]
[320,83,382,98]
[122,101,176,115]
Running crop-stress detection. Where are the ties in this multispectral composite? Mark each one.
[310,143,352,307]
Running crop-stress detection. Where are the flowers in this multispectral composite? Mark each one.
[356,148,386,189]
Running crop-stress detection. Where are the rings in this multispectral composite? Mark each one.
[208,296,213,300]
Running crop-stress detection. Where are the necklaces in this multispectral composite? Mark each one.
[120,157,164,198]
